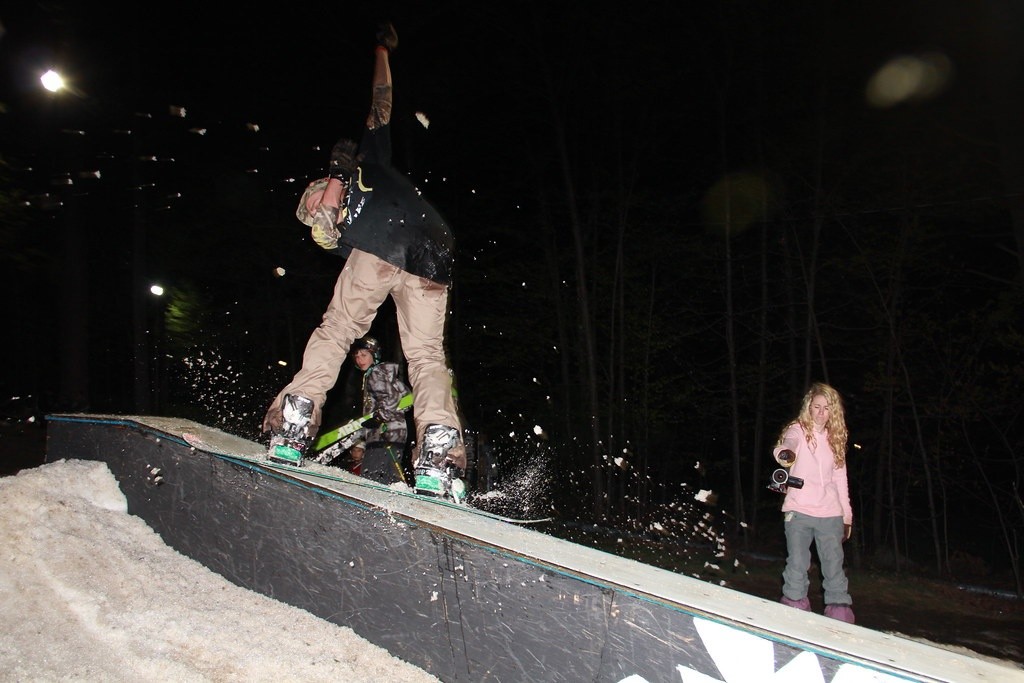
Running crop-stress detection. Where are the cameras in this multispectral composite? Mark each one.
[772,468,804,490]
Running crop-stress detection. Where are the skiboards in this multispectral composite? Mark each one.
[307,369,457,464]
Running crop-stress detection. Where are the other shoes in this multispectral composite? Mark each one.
[824,603,853,626]
[780,595,810,612]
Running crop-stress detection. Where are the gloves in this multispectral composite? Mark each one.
[360,413,384,429]
[311,204,341,249]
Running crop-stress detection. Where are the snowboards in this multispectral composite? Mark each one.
[182,431,556,525]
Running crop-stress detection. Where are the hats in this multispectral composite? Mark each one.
[296,178,330,227]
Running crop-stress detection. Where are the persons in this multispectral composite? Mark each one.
[345,440,366,475]
[263,23,467,502]
[352,337,411,485]
[773,384,855,625]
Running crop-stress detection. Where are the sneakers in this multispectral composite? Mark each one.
[267,393,315,467]
[414,423,465,499]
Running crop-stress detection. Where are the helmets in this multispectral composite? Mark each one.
[352,336,381,363]
[349,441,365,458]
